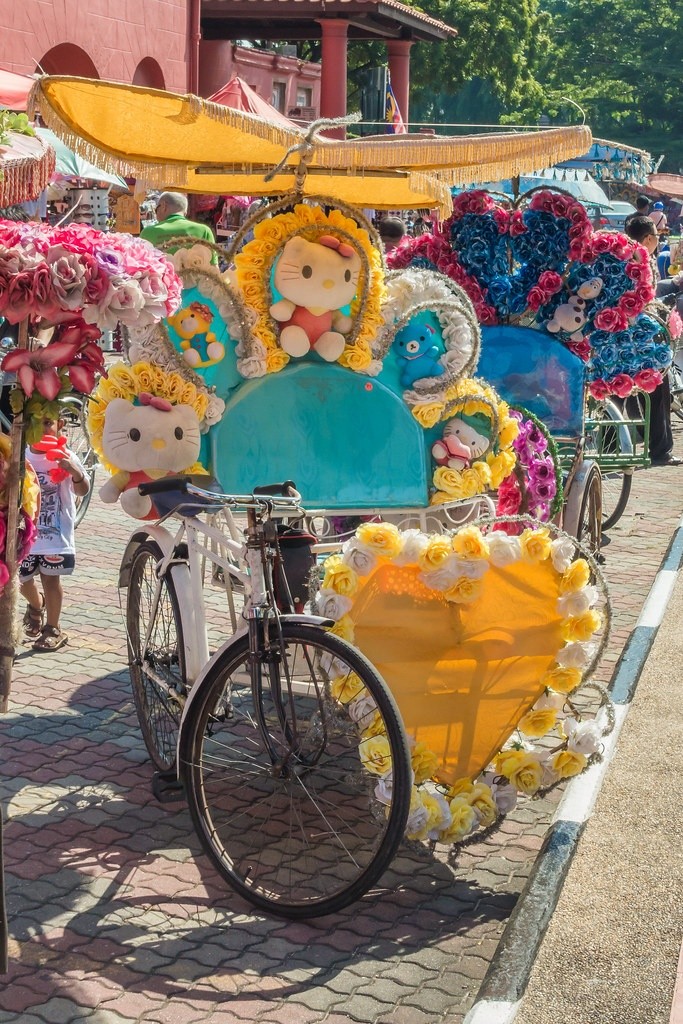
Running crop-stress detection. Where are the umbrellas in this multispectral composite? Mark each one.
[31,125,130,190]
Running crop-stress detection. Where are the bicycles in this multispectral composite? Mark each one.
[561,372,651,604]
[112,479,412,918]
[0,335,95,531]
[661,342,683,418]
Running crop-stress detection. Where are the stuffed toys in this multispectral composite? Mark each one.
[97,392,215,521]
[546,276,604,343]
[431,413,493,470]
[392,324,444,386]
[167,301,225,369]
[268,235,361,363]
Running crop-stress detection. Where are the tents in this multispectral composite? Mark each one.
[0,67,53,112]
[204,78,299,128]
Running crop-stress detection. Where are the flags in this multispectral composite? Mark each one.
[385,74,407,134]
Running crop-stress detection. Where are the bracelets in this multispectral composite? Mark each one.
[71,472,85,484]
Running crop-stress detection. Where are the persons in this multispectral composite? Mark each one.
[16,402,92,652]
[600,196,683,465]
[374,216,406,253]
[139,190,218,268]
[228,199,272,253]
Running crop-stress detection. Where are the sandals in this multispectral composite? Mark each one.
[32,625,68,651]
[651,456,683,465]
[21,592,47,637]
